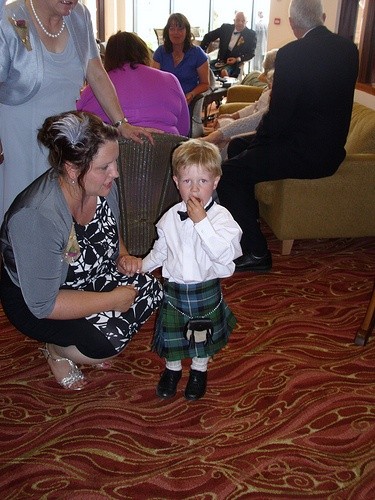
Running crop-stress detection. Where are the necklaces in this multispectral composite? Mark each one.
[30,0,65,38]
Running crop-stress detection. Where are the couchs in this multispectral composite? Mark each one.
[227,71,269,102]
[215,102,375,256]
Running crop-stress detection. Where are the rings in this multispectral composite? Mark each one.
[131,303,134,306]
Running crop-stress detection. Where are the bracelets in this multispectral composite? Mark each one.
[114,118,128,128]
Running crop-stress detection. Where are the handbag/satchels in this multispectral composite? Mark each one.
[183,318,216,348]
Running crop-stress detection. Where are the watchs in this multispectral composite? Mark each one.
[190,91,195,99]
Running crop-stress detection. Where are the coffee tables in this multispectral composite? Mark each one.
[203,75,241,127]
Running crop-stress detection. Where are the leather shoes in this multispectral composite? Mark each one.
[184,367,208,400]
[233,251,272,272]
[157,366,182,398]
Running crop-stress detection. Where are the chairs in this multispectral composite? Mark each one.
[115,133,187,255]
[154,28,164,45]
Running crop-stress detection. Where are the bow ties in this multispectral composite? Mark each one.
[234,32,242,35]
[177,199,214,221]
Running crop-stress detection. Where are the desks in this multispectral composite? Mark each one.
[192,37,218,53]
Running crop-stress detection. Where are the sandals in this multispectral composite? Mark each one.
[91,360,114,370]
[38,343,85,390]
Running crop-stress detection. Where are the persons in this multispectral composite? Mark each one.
[206,71,273,145]
[76,30,190,136]
[216,0,359,272]
[120,139,243,400]
[0,111,164,390]
[152,13,211,129]
[200,12,257,78]
[0,0,164,229]
